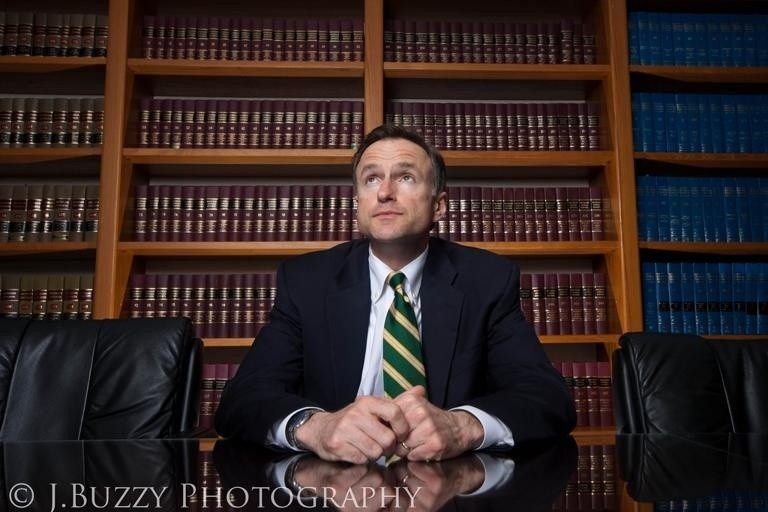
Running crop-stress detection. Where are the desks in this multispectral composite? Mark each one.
[0,445,762,509]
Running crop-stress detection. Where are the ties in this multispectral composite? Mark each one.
[382,274,429,467]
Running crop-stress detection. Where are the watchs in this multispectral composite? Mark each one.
[286,409,323,449]
[285,454,316,498]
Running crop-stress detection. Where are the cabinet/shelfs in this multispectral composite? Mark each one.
[0,3,766,511]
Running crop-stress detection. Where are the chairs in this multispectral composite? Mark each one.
[0,316,205,509]
[608,330,765,500]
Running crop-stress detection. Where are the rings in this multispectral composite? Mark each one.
[402,474,410,486]
[401,441,410,451]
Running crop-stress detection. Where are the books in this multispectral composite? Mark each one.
[549,445,620,511]
[180,450,237,512]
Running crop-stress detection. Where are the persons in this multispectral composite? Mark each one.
[211,434,578,512]
[213,123,576,466]
